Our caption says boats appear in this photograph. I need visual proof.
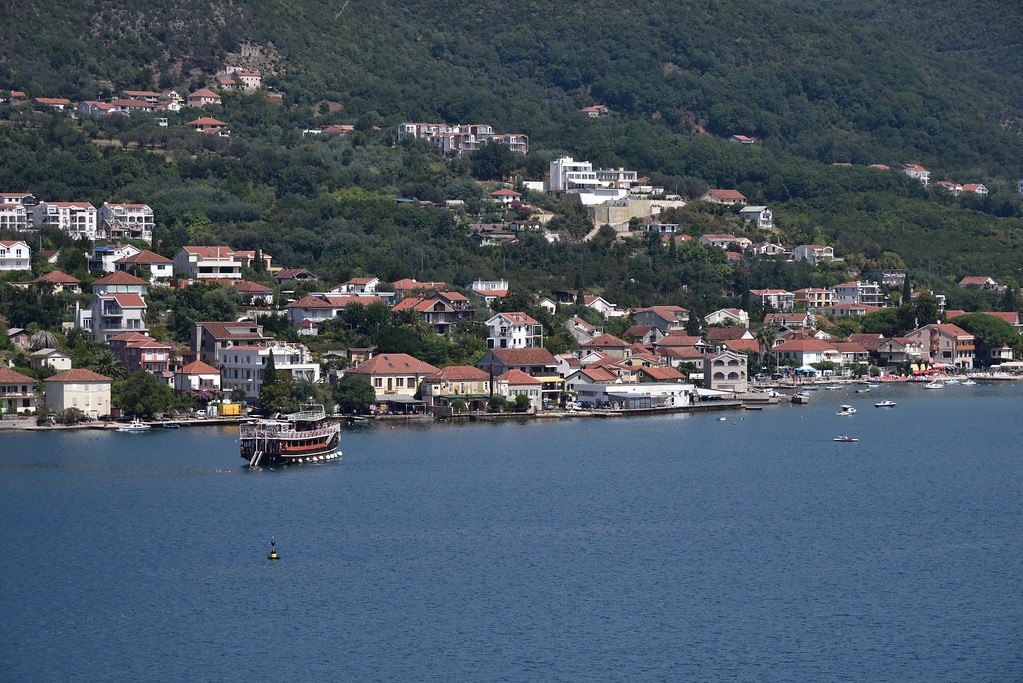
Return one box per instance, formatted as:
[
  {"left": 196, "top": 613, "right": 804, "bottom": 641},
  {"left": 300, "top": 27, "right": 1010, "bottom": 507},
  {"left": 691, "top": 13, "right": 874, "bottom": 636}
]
[
  {"left": 825, "top": 384, "right": 843, "bottom": 390},
  {"left": 834, "top": 434, "right": 859, "bottom": 442},
  {"left": 240, "top": 397, "right": 341, "bottom": 464},
  {"left": 836, "top": 404, "right": 856, "bottom": 416},
  {"left": 875, "top": 400, "right": 897, "bottom": 408},
  {"left": 923, "top": 373, "right": 976, "bottom": 389},
  {"left": 163, "top": 422, "right": 180, "bottom": 429},
  {"left": 791, "top": 380, "right": 818, "bottom": 404},
  {"left": 116, "top": 415, "right": 151, "bottom": 431},
  {"left": 868, "top": 384, "right": 880, "bottom": 388},
  {"left": 855, "top": 389, "right": 871, "bottom": 394}
]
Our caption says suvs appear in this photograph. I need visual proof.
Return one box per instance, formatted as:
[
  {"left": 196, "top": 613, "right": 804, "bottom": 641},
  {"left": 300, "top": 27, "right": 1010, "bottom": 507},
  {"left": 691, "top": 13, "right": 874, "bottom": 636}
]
[
  {"left": 47, "top": 414, "right": 57, "bottom": 425},
  {"left": 197, "top": 410, "right": 206, "bottom": 418}
]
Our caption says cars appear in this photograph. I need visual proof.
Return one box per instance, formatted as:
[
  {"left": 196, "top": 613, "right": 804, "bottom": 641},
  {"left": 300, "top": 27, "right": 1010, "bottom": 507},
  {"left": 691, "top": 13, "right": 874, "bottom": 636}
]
[
  {"left": 80, "top": 415, "right": 92, "bottom": 422},
  {"left": 755, "top": 373, "right": 784, "bottom": 381},
  {"left": 98, "top": 414, "right": 155, "bottom": 422},
  {"left": 559, "top": 400, "right": 594, "bottom": 410}
]
